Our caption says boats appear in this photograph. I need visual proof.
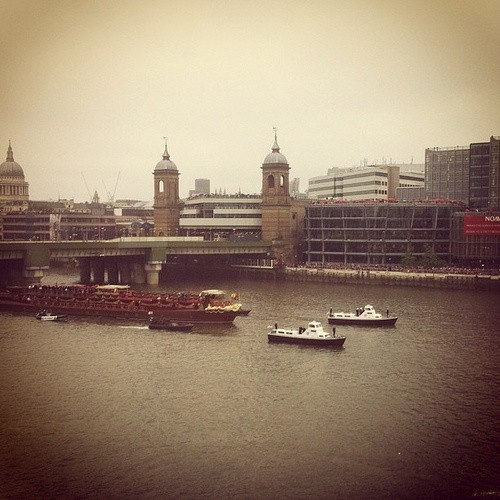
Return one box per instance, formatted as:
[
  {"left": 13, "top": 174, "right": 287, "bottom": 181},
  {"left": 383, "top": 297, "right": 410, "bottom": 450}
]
[
  {"left": 325, "top": 303, "right": 399, "bottom": 326},
  {"left": 0, "top": 282, "right": 243, "bottom": 324},
  {"left": 237, "top": 307, "right": 252, "bottom": 316},
  {"left": 33, "top": 311, "right": 69, "bottom": 321},
  {"left": 265, "top": 319, "right": 347, "bottom": 347},
  {"left": 147, "top": 311, "right": 195, "bottom": 331}
]
